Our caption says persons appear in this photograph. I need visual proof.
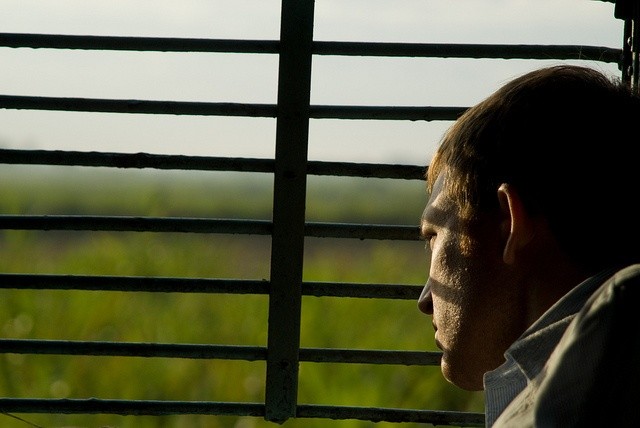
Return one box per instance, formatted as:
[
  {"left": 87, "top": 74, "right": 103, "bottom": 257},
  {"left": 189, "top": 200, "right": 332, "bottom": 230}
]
[{"left": 418, "top": 66, "right": 640, "bottom": 428}]
